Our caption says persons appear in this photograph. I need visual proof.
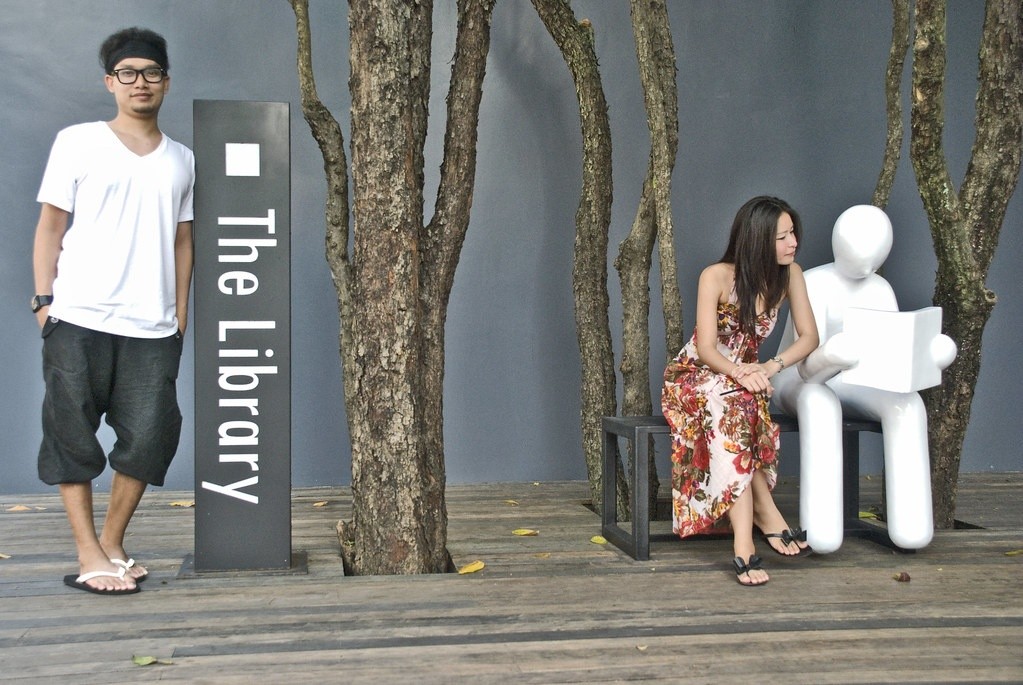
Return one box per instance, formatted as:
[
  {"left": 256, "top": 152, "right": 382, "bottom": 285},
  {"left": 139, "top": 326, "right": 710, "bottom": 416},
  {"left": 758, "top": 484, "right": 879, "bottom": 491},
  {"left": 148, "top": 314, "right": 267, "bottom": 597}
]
[
  {"left": 31, "top": 26, "right": 196, "bottom": 597},
  {"left": 770, "top": 204, "right": 957, "bottom": 555},
  {"left": 660, "top": 196, "right": 819, "bottom": 586}
]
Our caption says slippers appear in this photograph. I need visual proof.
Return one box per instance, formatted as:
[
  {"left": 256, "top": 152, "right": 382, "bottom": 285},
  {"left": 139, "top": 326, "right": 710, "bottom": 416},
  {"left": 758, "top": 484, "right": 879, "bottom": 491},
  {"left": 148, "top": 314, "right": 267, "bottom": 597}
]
[
  {"left": 64, "top": 571, "right": 140, "bottom": 594},
  {"left": 753, "top": 522, "right": 814, "bottom": 558},
  {"left": 733, "top": 556, "right": 768, "bottom": 586},
  {"left": 111, "top": 557, "right": 145, "bottom": 582}
]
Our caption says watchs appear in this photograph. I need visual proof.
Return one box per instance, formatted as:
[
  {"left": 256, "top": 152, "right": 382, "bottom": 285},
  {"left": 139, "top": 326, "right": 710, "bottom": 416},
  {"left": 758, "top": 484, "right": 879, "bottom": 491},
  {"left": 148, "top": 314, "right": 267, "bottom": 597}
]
[
  {"left": 31, "top": 295, "right": 53, "bottom": 313},
  {"left": 771, "top": 356, "right": 783, "bottom": 373}
]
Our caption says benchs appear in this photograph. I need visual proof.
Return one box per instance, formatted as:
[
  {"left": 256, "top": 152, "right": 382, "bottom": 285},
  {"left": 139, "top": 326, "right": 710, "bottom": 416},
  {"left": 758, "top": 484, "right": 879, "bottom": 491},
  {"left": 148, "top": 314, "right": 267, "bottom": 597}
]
[{"left": 601, "top": 413, "right": 915, "bottom": 563}]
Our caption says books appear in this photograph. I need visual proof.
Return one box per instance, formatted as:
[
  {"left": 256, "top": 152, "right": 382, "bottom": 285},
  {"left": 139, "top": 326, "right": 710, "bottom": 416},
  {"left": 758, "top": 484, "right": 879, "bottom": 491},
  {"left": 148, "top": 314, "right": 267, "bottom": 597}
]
[{"left": 842, "top": 305, "right": 942, "bottom": 393}]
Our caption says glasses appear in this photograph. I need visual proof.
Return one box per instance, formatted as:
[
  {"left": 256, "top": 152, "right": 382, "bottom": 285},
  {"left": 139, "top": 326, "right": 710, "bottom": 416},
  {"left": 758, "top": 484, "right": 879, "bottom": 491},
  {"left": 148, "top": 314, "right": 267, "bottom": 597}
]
[{"left": 109, "top": 66, "right": 165, "bottom": 85}]
